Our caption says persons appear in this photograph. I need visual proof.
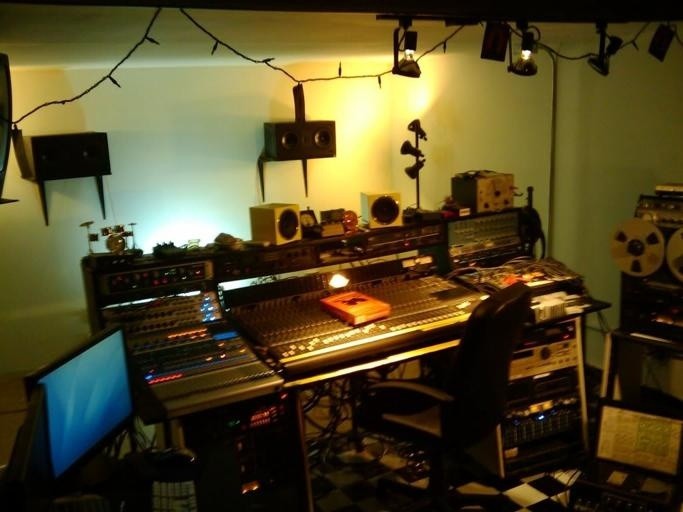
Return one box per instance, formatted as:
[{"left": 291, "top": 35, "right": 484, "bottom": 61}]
[{"left": 496, "top": 269, "right": 548, "bottom": 284}]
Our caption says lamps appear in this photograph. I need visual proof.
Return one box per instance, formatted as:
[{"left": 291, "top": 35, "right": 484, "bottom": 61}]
[{"left": 384, "top": 10, "right": 623, "bottom": 79}]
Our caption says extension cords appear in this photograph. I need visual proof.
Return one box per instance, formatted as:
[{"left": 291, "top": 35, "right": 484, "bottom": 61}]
[{"left": 337, "top": 449, "right": 375, "bottom": 463}]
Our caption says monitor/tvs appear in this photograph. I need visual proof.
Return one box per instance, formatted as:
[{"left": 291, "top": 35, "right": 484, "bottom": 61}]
[
  {"left": 593, "top": 398, "right": 683, "bottom": 478},
  {"left": 22, "top": 321, "right": 140, "bottom": 477}
]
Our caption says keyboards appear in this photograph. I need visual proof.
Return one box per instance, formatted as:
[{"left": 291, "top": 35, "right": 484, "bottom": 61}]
[{"left": 152, "top": 480, "right": 197, "bottom": 512}]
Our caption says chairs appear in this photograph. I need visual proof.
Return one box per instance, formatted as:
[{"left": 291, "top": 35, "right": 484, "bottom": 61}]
[{"left": 358, "top": 280, "right": 532, "bottom": 511}]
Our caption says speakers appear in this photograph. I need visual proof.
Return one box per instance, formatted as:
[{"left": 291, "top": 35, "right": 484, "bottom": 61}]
[
  {"left": 360, "top": 192, "right": 403, "bottom": 229},
  {"left": 248, "top": 202, "right": 302, "bottom": 246},
  {"left": 263, "top": 120, "right": 337, "bottom": 161},
  {"left": 25, "top": 129, "right": 113, "bottom": 183}
]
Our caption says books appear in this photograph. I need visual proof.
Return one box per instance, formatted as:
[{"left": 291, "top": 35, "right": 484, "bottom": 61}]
[
  {"left": 446, "top": 257, "right": 584, "bottom": 289},
  {"left": 319, "top": 290, "right": 392, "bottom": 325}
]
